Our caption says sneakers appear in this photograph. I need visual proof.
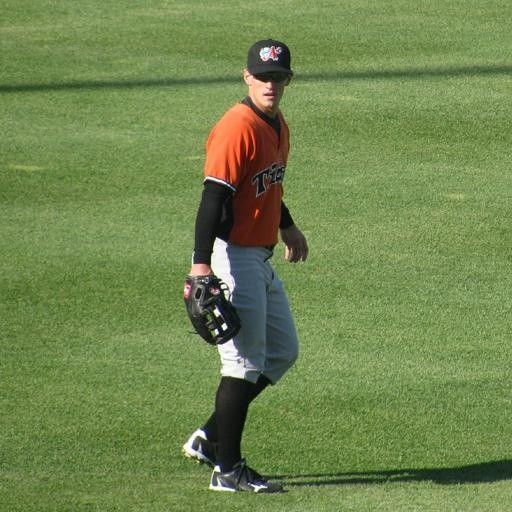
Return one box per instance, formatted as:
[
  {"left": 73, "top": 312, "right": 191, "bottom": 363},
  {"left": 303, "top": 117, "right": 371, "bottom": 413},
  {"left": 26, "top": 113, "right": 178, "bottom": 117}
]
[
  {"left": 207, "top": 457, "right": 283, "bottom": 495},
  {"left": 180, "top": 427, "right": 215, "bottom": 471}
]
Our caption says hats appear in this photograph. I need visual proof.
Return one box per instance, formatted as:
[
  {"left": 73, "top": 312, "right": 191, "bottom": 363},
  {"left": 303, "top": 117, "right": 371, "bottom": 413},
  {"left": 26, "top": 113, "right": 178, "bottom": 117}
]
[{"left": 247, "top": 39, "right": 293, "bottom": 76}]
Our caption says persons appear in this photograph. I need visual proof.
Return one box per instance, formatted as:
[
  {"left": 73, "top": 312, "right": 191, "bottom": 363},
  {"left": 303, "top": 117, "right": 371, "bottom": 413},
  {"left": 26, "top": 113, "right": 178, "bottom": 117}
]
[{"left": 181, "top": 39, "right": 309, "bottom": 493}]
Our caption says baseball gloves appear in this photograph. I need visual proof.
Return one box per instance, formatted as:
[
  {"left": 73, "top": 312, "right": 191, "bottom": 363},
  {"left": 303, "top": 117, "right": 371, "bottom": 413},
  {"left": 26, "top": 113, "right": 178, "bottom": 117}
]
[{"left": 183, "top": 276, "right": 241, "bottom": 344}]
